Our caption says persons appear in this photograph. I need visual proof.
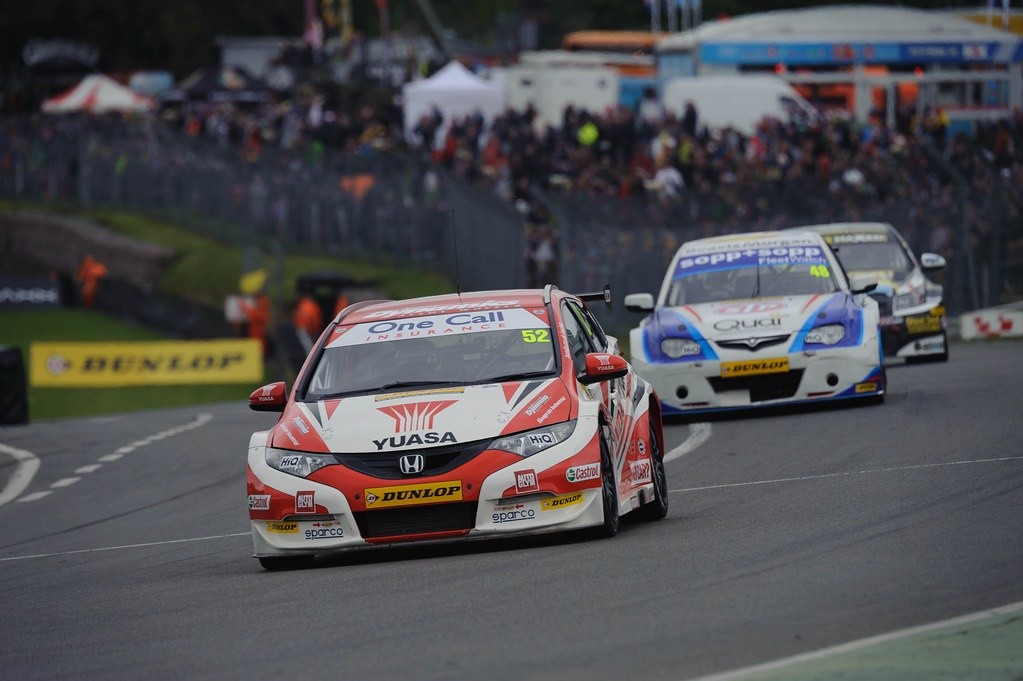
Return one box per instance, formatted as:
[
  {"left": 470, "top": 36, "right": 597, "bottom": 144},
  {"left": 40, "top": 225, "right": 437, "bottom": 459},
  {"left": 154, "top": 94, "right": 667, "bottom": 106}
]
[{"left": 0, "top": 40, "right": 1023, "bottom": 358}]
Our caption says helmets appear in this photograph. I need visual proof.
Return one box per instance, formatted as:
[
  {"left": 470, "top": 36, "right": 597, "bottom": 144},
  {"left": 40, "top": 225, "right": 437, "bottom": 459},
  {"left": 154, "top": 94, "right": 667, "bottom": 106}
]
[
  {"left": 395, "top": 339, "right": 442, "bottom": 380},
  {"left": 703, "top": 269, "right": 737, "bottom": 300}
]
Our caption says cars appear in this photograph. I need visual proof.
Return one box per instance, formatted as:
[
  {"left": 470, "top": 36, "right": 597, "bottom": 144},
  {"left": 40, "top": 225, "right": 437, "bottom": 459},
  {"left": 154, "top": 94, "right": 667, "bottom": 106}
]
[
  {"left": 246, "top": 284, "right": 668, "bottom": 572},
  {"left": 624, "top": 229, "right": 889, "bottom": 417},
  {"left": 788, "top": 221, "right": 952, "bottom": 365}
]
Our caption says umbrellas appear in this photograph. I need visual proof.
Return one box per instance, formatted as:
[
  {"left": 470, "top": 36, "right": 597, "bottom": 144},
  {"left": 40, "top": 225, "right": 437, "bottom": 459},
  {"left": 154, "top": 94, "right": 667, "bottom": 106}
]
[{"left": 40, "top": 74, "right": 162, "bottom": 114}]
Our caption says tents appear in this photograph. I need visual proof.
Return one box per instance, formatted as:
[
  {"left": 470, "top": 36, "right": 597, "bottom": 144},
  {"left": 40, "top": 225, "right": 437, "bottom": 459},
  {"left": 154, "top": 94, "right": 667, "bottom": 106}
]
[{"left": 401, "top": 59, "right": 506, "bottom": 148}]
